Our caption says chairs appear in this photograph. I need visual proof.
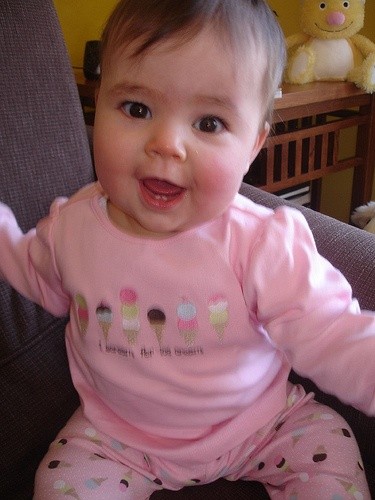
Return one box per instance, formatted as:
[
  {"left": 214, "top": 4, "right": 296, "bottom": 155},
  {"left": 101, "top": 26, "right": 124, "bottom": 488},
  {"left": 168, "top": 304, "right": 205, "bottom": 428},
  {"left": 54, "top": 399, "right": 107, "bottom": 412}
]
[{"left": 0, "top": 0, "right": 375, "bottom": 500}]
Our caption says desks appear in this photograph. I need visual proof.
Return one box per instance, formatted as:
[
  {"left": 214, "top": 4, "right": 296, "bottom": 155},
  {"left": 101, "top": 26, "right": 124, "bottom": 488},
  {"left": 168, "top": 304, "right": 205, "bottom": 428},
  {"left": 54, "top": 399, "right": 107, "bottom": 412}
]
[{"left": 73, "top": 68, "right": 375, "bottom": 230}]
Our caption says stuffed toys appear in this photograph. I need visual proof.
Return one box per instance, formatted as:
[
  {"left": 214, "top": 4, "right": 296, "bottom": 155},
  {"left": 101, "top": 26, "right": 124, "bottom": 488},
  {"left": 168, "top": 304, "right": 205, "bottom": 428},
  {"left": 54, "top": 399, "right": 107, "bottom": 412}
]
[
  {"left": 351, "top": 201, "right": 375, "bottom": 234},
  {"left": 284, "top": 0, "right": 375, "bottom": 94}
]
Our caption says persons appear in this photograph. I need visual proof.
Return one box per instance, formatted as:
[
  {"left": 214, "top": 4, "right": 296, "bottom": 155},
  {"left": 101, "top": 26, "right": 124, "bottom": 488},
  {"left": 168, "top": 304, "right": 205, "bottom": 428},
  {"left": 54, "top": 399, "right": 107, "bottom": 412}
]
[{"left": 0, "top": 0, "right": 375, "bottom": 500}]
